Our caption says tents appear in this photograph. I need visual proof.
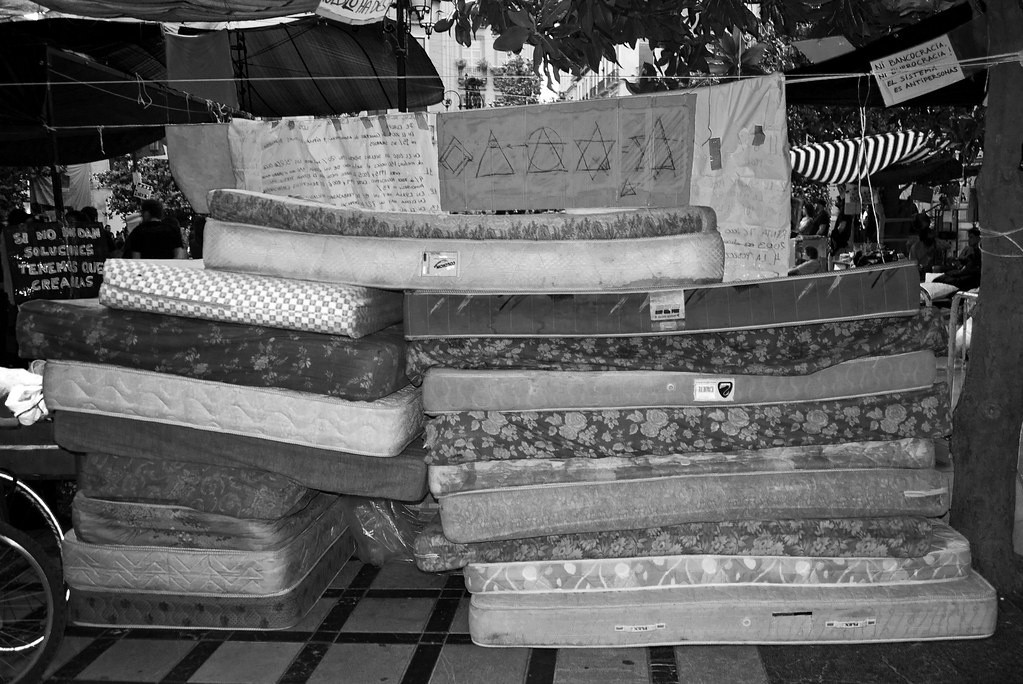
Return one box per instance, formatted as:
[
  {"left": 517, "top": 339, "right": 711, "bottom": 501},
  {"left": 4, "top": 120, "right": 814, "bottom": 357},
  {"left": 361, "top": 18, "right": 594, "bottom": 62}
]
[
  {"left": 0, "top": 0, "right": 445, "bottom": 220},
  {"left": 789, "top": 131, "right": 965, "bottom": 226}
]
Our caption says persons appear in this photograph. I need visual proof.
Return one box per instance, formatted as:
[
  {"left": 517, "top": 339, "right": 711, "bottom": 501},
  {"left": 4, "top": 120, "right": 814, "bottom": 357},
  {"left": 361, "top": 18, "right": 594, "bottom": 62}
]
[
  {"left": 790, "top": 195, "right": 982, "bottom": 307},
  {"left": 2, "top": 201, "right": 206, "bottom": 260}
]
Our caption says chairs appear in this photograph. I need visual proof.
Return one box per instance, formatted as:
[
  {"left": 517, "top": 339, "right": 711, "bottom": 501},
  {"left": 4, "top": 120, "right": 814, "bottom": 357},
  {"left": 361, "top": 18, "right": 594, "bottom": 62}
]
[{"left": 934, "top": 208, "right": 958, "bottom": 261}]
